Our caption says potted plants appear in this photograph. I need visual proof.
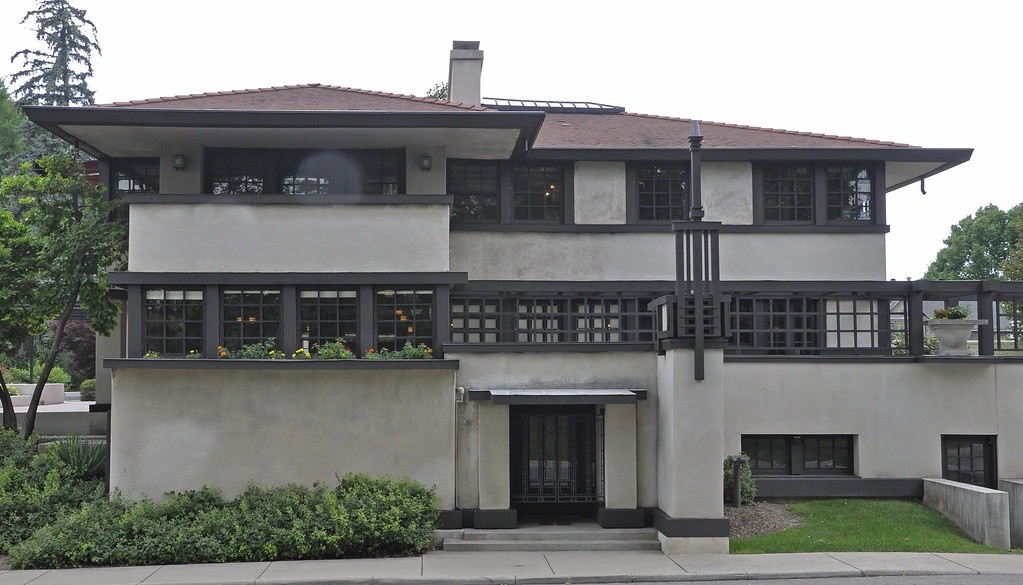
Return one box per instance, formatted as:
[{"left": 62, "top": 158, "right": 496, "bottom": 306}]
[{"left": 928, "top": 304, "right": 988, "bottom": 355}]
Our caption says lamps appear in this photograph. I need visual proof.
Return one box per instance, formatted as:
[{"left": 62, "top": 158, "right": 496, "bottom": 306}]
[
  {"left": 420, "top": 154, "right": 432, "bottom": 171},
  {"left": 172, "top": 153, "right": 185, "bottom": 170}
]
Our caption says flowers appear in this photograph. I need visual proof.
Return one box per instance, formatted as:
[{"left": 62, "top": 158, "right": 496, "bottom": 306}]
[{"left": 145, "top": 336, "right": 433, "bottom": 360}]
